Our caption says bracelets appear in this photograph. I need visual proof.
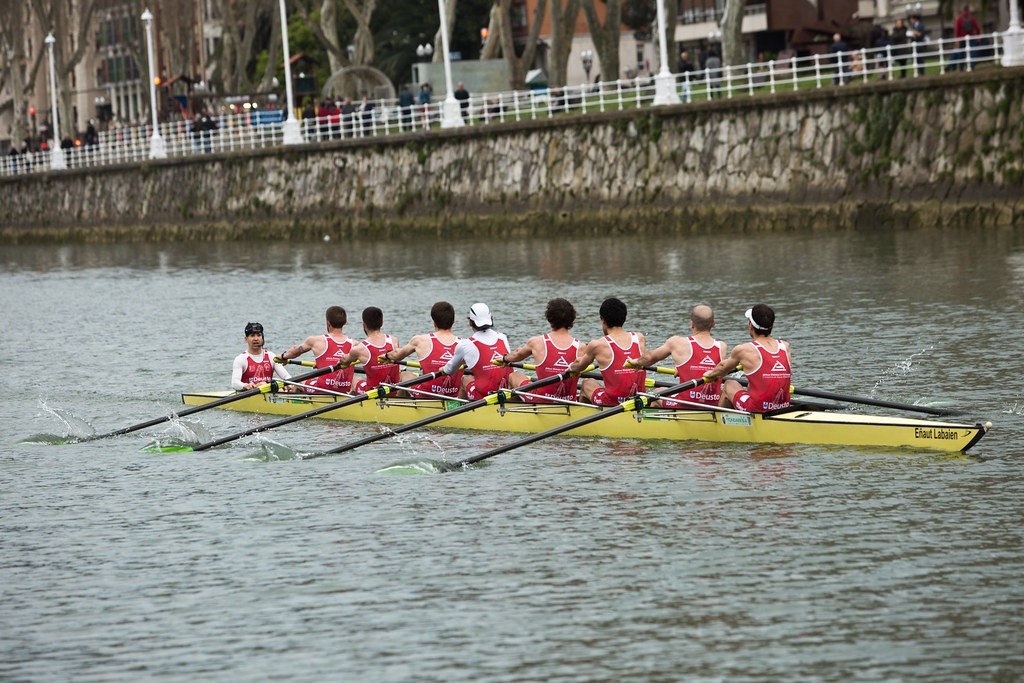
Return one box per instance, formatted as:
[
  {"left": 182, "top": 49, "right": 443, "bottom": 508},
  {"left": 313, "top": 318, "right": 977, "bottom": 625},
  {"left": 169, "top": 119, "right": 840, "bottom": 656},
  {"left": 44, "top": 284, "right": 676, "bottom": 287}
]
[
  {"left": 386, "top": 350, "right": 392, "bottom": 359},
  {"left": 503, "top": 353, "right": 508, "bottom": 363},
  {"left": 568, "top": 362, "right": 573, "bottom": 371},
  {"left": 282, "top": 351, "right": 287, "bottom": 360}
]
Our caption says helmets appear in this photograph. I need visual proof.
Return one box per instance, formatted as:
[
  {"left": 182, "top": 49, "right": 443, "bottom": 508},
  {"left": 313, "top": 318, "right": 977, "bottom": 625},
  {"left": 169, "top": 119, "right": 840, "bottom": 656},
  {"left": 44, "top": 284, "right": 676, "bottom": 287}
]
[{"left": 245, "top": 322, "right": 263, "bottom": 337}]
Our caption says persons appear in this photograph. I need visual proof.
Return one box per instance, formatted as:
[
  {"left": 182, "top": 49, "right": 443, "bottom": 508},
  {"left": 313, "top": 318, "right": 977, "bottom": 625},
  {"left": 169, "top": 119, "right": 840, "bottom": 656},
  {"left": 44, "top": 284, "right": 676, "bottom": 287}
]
[
  {"left": 547, "top": 85, "right": 564, "bottom": 113},
  {"left": 193, "top": 112, "right": 216, "bottom": 152},
  {"left": 401, "top": 82, "right": 432, "bottom": 130},
  {"left": 455, "top": 81, "right": 469, "bottom": 122},
  {"left": 302, "top": 96, "right": 372, "bottom": 140},
  {"left": 627, "top": 305, "right": 727, "bottom": 410},
  {"left": 336, "top": 307, "right": 400, "bottom": 397},
  {"left": 496, "top": 298, "right": 586, "bottom": 404},
  {"left": 377, "top": 301, "right": 466, "bottom": 398},
  {"left": 9, "top": 141, "right": 28, "bottom": 175},
  {"left": 439, "top": 303, "right": 514, "bottom": 401},
  {"left": 565, "top": 298, "right": 646, "bottom": 405},
  {"left": 830, "top": 34, "right": 863, "bottom": 84},
  {"left": 678, "top": 49, "right": 721, "bottom": 101},
  {"left": 478, "top": 98, "right": 509, "bottom": 120},
  {"left": 232, "top": 322, "right": 292, "bottom": 393},
  {"left": 85, "top": 120, "right": 95, "bottom": 150},
  {"left": 955, "top": 5, "right": 981, "bottom": 70},
  {"left": 869, "top": 16, "right": 926, "bottom": 80},
  {"left": 61, "top": 133, "right": 75, "bottom": 165},
  {"left": 276, "top": 306, "right": 359, "bottom": 393},
  {"left": 704, "top": 303, "right": 793, "bottom": 414}
]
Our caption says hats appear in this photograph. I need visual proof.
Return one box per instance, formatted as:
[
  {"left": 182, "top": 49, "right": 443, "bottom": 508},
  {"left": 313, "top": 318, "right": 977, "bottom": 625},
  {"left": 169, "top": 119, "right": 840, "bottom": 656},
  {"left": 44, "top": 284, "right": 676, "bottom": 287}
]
[
  {"left": 745, "top": 309, "right": 771, "bottom": 329},
  {"left": 470, "top": 302, "right": 493, "bottom": 326}
]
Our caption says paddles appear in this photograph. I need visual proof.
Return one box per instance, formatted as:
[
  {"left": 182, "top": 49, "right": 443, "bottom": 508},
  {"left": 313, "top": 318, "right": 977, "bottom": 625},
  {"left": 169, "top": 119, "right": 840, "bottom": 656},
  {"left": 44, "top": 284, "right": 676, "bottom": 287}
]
[
  {"left": 271, "top": 357, "right": 315, "bottom": 367},
  {"left": 373, "top": 363, "right": 743, "bottom": 475},
  {"left": 491, "top": 358, "right": 678, "bottom": 388},
  {"left": 623, "top": 360, "right": 970, "bottom": 416},
  {"left": 297, "top": 361, "right": 601, "bottom": 461},
  {"left": 55, "top": 359, "right": 359, "bottom": 445},
  {"left": 375, "top": 357, "right": 422, "bottom": 368},
  {"left": 148, "top": 362, "right": 466, "bottom": 454}
]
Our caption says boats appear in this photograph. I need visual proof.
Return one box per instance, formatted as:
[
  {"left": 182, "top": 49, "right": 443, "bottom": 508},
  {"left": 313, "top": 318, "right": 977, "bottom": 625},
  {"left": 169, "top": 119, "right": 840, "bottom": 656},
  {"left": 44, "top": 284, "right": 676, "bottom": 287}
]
[{"left": 182, "top": 391, "right": 993, "bottom": 452}]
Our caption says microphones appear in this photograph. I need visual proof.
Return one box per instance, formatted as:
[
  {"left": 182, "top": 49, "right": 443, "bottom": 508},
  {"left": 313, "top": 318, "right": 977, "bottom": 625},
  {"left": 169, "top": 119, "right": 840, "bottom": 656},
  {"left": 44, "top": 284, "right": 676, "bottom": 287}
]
[{"left": 258, "top": 334, "right": 265, "bottom": 348}]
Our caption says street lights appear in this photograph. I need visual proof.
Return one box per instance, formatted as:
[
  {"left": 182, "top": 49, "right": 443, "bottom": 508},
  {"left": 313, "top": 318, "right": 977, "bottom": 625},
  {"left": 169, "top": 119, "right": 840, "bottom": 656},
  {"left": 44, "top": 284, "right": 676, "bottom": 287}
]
[
  {"left": 43, "top": 32, "right": 68, "bottom": 170},
  {"left": 140, "top": 7, "right": 167, "bottom": 160},
  {"left": 580, "top": 50, "right": 593, "bottom": 82}
]
